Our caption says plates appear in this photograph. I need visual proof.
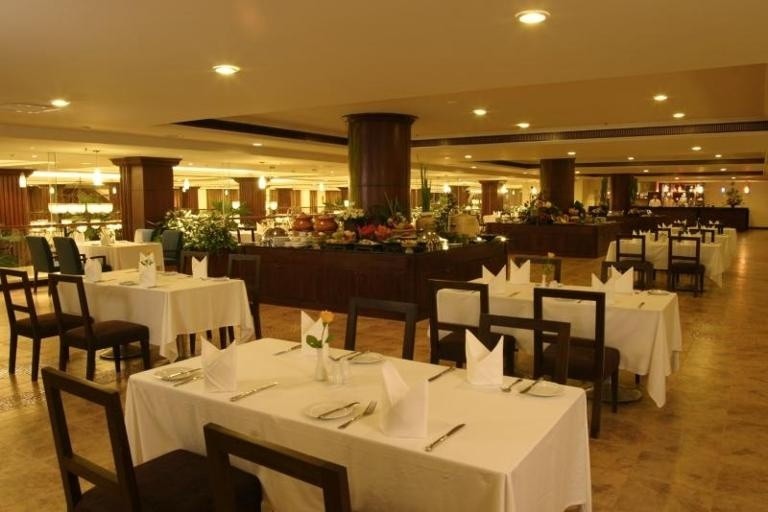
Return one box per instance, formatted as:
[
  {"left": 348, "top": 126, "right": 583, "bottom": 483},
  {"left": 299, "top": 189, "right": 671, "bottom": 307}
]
[
  {"left": 344, "top": 352, "right": 384, "bottom": 365},
  {"left": 472, "top": 239, "right": 487, "bottom": 244},
  {"left": 153, "top": 366, "right": 197, "bottom": 380},
  {"left": 520, "top": 380, "right": 563, "bottom": 398},
  {"left": 303, "top": 401, "right": 352, "bottom": 420}
]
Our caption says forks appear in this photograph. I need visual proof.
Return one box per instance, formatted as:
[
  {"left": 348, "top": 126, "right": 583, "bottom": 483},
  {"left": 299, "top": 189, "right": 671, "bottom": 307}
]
[
  {"left": 500, "top": 378, "right": 524, "bottom": 393},
  {"left": 326, "top": 351, "right": 356, "bottom": 362},
  {"left": 337, "top": 402, "right": 378, "bottom": 430}
]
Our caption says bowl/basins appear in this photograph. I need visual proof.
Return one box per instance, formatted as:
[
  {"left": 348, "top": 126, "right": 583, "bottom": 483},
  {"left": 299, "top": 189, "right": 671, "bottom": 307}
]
[{"left": 479, "top": 234, "right": 497, "bottom": 242}]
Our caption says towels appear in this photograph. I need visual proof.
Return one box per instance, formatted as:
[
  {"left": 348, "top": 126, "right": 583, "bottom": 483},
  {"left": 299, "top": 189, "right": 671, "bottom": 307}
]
[
  {"left": 197, "top": 336, "right": 238, "bottom": 394},
  {"left": 299, "top": 311, "right": 328, "bottom": 353},
  {"left": 372, "top": 355, "right": 429, "bottom": 442}
]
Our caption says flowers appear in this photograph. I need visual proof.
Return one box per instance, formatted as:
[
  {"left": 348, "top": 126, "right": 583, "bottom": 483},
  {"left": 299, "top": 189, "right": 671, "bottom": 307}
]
[
  {"left": 335, "top": 207, "right": 367, "bottom": 223},
  {"left": 306, "top": 311, "right": 336, "bottom": 348}
]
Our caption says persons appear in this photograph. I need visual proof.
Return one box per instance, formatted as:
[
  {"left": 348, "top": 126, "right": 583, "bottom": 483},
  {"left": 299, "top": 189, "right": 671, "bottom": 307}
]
[{"left": 647, "top": 195, "right": 660, "bottom": 207}]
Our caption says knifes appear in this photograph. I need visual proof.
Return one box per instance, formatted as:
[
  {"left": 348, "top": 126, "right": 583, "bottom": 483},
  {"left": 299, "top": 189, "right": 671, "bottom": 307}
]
[
  {"left": 173, "top": 375, "right": 201, "bottom": 387},
  {"left": 508, "top": 291, "right": 518, "bottom": 298},
  {"left": 428, "top": 365, "right": 455, "bottom": 384},
  {"left": 316, "top": 400, "right": 359, "bottom": 420},
  {"left": 636, "top": 301, "right": 645, "bottom": 309},
  {"left": 423, "top": 424, "right": 464, "bottom": 455},
  {"left": 228, "top": 381, "right": 278, "bottom": 403},
  {"left": 518, "top": 376, "right": 543, "bottom": 395},
  {"left": 273, "top": 342, "right": 303, "bottom": 356},
  {"left": 94, "top": 270, "right": 228, "bottom": 290},
  {"left": 168, "top": 365, "right": 201, "bottom": 379}
]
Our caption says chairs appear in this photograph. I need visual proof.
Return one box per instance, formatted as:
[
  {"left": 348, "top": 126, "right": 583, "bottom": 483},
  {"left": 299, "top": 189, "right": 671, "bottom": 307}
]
[
  {"left": 37, "top": 366, "right": 263, "bottom": 512},
  {"left": 203, "top": 422, "right": 351, "bottom": 512},
  {"left": 343, "top": 297, "right": 419, "bottom": 359}
]
